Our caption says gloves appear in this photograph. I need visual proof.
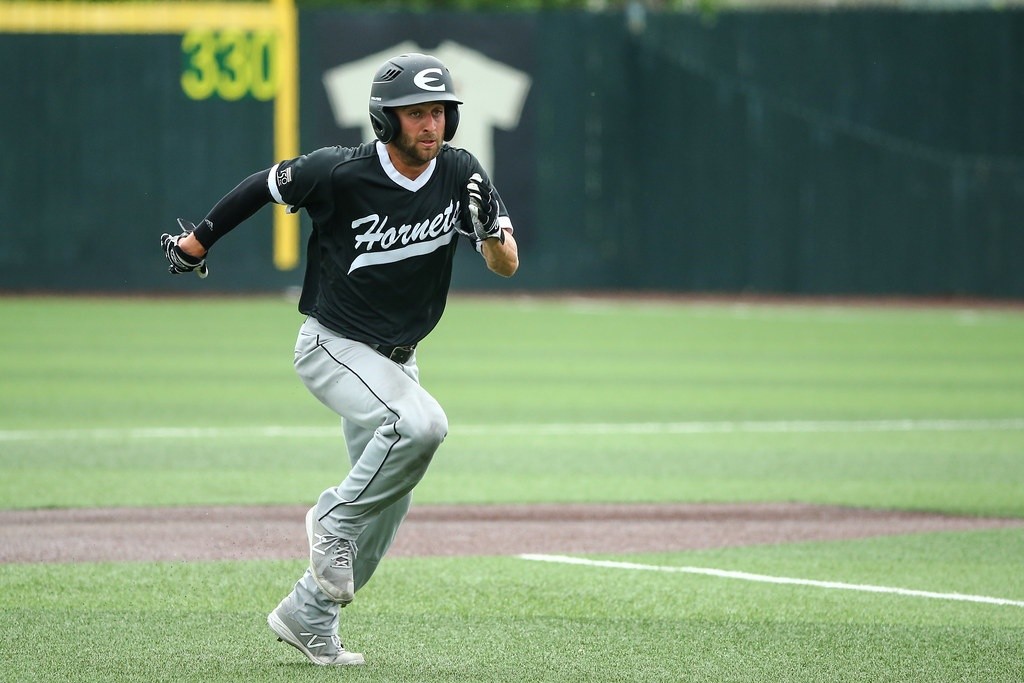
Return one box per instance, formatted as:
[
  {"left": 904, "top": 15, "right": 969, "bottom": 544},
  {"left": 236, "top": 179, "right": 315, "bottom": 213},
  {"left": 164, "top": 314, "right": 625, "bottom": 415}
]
[
  {"left": 161, "top": 218, "right": 209, "bottom": 279},
  {"left": 467, "top": 173, "right": 505, "bottom": 244}
]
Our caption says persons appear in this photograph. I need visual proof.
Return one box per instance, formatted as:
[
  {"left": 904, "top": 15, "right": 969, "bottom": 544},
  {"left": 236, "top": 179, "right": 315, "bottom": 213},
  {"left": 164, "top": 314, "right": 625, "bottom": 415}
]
[{"left": 159, "top": 53, "right": 519, "bottom": 667}]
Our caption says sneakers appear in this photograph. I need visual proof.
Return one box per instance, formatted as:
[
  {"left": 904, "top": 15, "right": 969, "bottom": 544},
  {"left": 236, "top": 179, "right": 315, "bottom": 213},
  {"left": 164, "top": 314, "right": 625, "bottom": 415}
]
[
  {"left": 306, "top": 505, "right": 354, "bottom": 601},
  {"left": 267, "top": 597, "right": 364, "bottom": 665}
]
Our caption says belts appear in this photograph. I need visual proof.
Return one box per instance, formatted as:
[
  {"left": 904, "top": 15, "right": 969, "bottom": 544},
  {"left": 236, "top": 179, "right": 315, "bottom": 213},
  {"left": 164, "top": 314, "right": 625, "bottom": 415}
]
[{"left": 370, "top": 342, "right": 417, "bottom": 364}]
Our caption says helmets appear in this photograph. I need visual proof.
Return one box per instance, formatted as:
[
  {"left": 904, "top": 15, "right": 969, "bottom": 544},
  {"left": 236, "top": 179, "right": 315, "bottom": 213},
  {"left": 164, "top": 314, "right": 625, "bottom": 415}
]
[{"left": 369, "top": 53, "right": 463, "bottom": 144}]
